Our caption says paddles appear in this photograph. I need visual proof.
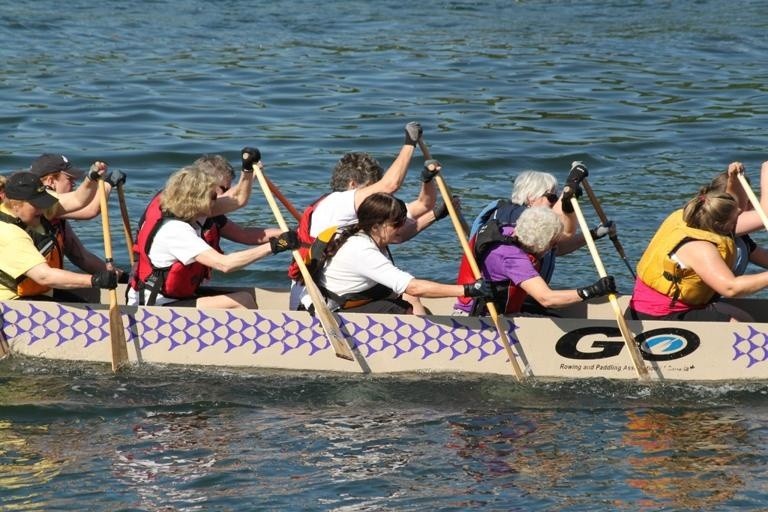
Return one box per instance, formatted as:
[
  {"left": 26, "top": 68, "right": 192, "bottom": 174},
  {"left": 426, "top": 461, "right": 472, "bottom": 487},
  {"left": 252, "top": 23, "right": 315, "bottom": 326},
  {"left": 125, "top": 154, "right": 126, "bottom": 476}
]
[
  {"left": 427, "top": 159, "right": 525, "bottom": 382},
  {"left": 92, "top": 158, "right": 130, "bottom": 373},
  {"left": 559, "top": 185, "right": 650, "bottom": 380},
  {"left": 240, "top": 153, "right": 356, "bottom": 363}
]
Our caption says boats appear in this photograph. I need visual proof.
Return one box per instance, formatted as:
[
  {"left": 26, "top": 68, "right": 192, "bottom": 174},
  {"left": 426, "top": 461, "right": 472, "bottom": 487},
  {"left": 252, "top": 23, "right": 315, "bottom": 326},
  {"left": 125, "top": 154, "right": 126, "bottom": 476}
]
[{"left": 1, "top": 276, "right": 768, "bottom": 385}]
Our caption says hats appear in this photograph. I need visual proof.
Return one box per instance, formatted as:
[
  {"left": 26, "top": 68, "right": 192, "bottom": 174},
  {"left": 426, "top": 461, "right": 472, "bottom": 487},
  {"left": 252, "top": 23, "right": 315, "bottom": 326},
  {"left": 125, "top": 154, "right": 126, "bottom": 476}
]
[
  {"left": 30, "top": 155, "right": 85, "bottom": 179},
  {"left": 4, "top": 173, "right": 58, "bottom": 209}
]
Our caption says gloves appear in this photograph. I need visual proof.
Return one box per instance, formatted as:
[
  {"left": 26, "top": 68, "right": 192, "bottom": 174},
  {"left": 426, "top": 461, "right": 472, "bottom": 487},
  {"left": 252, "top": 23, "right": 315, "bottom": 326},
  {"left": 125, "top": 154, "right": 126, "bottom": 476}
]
[
  {"left": 561, "top": 183, "right": 583, "bottom": 213},
  {"left": 464, "top": 278, "right": 494, "bottom": 297},
  {"left": 241, "top": 148, "right": 260, "bottom": 173},
  {"left": 269, "top": 231, "right": 300, "bottom": 255},
  {"left": 577, "top": 276, "right": 616, "bottom": 302},
  {"left": 433, "top": 198, "right": 460, "bottom": 221}
]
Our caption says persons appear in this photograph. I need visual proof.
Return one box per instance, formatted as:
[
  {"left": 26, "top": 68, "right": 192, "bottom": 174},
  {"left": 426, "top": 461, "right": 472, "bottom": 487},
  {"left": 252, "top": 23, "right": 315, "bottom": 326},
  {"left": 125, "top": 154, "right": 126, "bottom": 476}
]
[
  {"left": 696, "top": 162, "right": 768, "bottom": 302},
  {"left": 296, "top": 192, "right": 496, "bottom": 314},
  {"left": 125, "top": 147, "right": 301, "bottom": 309},
  {"left": 133, "top": 154, "right": 291, "bottom": 298},
  {"left": 286, "top": 121, "right": 442, "bottom": 311},
  {"left": 629, "top": 190, "right": 768, "bottom": 322},
  {"left": 468, "top": 183, "right": 616, "bottom": 317},
  {"left": 469, "top": 164, "right": 615, "bottom": 309},
  {"left": 0, "top": 160, "right": 118, "bottom": 301},
  {"left": 17, "top": 152, "right": 130, "bottom": 303}
]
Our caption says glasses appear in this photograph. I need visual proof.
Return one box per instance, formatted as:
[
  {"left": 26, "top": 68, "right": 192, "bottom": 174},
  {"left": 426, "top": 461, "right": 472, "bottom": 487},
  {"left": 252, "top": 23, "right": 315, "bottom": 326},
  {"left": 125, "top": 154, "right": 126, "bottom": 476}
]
[{"left": 543, "top": 191, "right": 558, "bottom": 203}]
